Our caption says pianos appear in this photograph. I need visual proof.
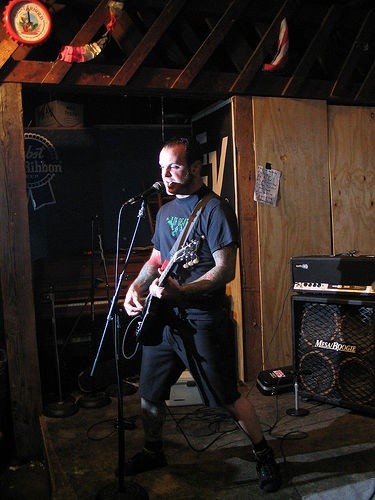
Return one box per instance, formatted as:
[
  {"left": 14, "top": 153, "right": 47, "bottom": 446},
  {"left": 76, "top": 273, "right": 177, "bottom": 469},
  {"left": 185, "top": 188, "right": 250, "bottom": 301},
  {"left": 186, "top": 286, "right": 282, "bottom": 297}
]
[{"left": 44, "top": 244, "right": 152, "bottom": 403}]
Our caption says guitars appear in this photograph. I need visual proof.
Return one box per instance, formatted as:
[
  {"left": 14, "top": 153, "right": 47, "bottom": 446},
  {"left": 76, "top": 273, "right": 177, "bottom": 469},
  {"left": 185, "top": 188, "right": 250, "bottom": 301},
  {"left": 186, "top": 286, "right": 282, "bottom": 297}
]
[{"left": 128, "top": 234, "right": 202, "bottom": 345}]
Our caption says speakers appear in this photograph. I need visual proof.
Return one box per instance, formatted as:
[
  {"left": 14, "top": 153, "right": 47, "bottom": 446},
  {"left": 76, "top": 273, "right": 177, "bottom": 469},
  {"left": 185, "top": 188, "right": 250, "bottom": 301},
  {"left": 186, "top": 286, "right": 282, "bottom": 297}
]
[{"left": 292, "top": 295, "right": 375, "bottom": 419}]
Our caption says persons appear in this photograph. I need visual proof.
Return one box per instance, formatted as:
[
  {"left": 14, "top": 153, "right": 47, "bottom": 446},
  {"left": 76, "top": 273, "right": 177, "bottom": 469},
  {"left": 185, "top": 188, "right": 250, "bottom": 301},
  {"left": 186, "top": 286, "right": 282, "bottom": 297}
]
[{"left": 114, "top": 138, "right": 278, "bottom": 490}]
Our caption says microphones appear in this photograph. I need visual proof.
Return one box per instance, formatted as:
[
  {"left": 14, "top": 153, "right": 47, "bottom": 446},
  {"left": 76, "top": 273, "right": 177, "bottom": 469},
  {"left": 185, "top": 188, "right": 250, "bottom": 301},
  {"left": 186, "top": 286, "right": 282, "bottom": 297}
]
[{"left": 128, "top": 181, "right": 164, "bottom": 205}]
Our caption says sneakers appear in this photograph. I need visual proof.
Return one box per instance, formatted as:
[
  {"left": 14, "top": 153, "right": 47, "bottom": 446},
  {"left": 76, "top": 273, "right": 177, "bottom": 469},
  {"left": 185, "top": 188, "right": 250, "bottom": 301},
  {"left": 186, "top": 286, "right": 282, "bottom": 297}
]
[
  {"left": 115, "top": 448, "right": 168, "bottom": 475},
  {"left": 252, "top": 440, "right": 280, "bottom": 492}
]
